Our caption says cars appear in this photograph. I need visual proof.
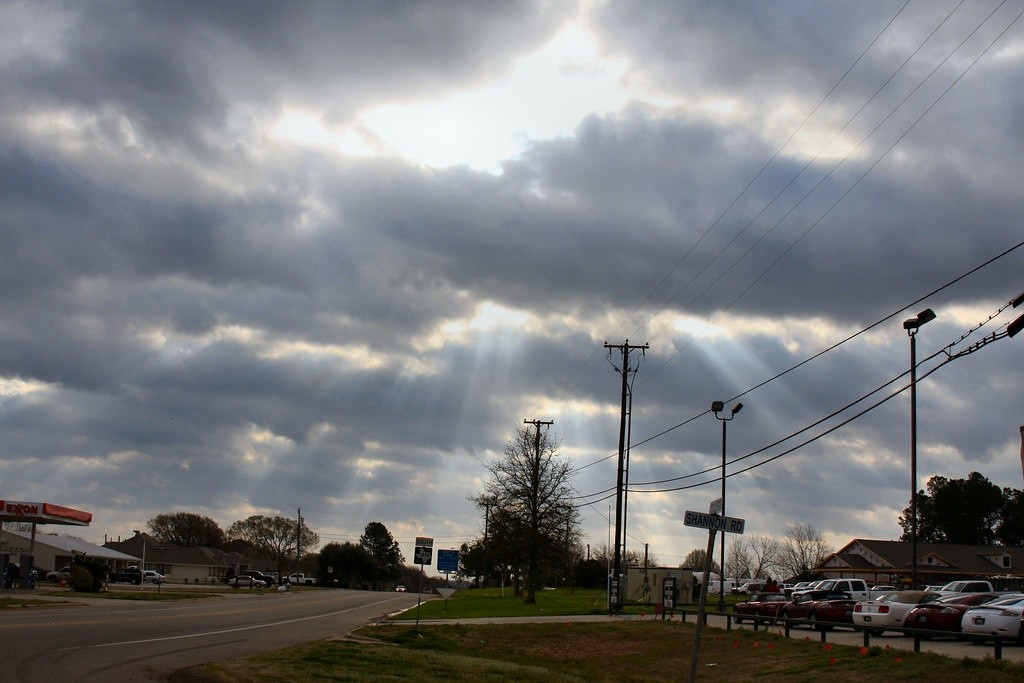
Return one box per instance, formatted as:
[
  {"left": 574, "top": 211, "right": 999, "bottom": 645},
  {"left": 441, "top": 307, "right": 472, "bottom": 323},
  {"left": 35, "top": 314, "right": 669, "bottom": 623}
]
[
  {"left": 395, "top": 586, "right": 408, "bottom": 592},
  {"left": 963, "top": 593, "right": 1024, "bottom": 646},
  {"left": 733, "top": 578, "right": 1024, "bottom": 613},
  {"left": 140, "top": 569, "right": 167, "bottom": 585},
  {"left": 732, "top": 592, "right": 793, "bottom": 626},
  {"left": 229, "top": 575, "right": 267, "bottom": 588},
  {"left": 852, "top": 589, "right": 942, "bottom": 637},
  {"left": 47, "top": 565, "right": 72, "bottom": 583},
  {"left": 904, "top": 594, "right": 1000, "bottom": 642},
  {"left": 781, "top": 589, "right": 860, "bottom": 632}
]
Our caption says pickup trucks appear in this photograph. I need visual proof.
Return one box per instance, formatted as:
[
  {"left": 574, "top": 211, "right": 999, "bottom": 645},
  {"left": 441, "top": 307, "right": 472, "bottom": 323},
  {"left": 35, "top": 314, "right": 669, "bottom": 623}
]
[{"left": 287, "top": 573, "right": 318, "bottom": 586}]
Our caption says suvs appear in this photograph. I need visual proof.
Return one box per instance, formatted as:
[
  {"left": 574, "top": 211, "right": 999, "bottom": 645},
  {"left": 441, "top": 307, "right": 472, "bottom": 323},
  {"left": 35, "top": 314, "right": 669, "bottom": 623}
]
[
  {"left": 108, "top": 567, "right": 145, "bottom": 586},
  {"left": 237, "top": 570, "right": 274, "bottom": 589}
]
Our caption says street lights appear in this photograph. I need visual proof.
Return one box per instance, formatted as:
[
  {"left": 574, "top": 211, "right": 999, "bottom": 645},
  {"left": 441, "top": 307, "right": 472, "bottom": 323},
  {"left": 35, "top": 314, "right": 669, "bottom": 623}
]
[
  {"left": 903, "top": 308, "right": 937, "bottom": 590},
  {"left": 712, "top": 400, "right": 744, "bottom": 612}
]
